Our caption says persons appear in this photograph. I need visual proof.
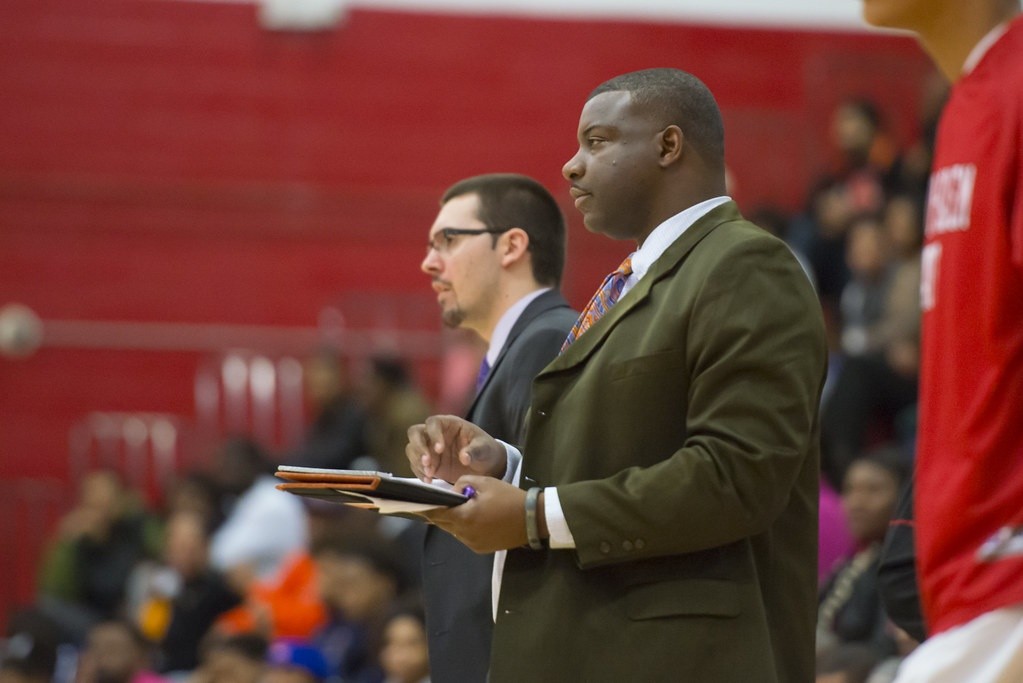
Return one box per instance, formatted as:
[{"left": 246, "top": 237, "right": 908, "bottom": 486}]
[
  {"left": 404, "top": 69, "right": 828, "bottom": 682},
  {"left": 418, "top": 174, "right": 581, "bottom": 683},
  {"left": 750, "top": 70, "right": 951, "bottom": 683},
  {"left": 0, "top": 347, "right": 432, "bottom": 683},
  {"left": 861, "top": 0, "right": 1023, "bottom": 682}
]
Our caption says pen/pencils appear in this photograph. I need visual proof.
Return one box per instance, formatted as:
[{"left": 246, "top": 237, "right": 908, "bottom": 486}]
[{"left": 463, "top": 486, "right": 475, "bottom": 498}]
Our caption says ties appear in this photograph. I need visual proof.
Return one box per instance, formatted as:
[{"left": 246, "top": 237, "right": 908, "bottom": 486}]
[{"left": 558, "top": 251, "right": 638, "bottom": 356}]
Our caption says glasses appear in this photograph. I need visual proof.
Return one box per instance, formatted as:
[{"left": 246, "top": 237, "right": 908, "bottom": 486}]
[{"left": 425, "top": 226, "right": 534, "bottom": 258}]
